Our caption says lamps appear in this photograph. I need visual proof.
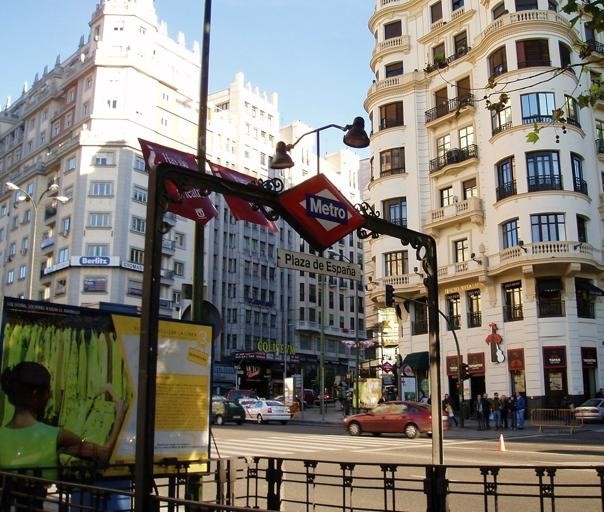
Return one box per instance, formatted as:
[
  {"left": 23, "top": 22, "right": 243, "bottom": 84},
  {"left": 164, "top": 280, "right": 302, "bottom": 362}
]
[
  {"left": 470, "top": 253, "right": 481, "bottom": 265},
  {"left": 518, "top": 240, "right": 528, "bottom": 254},
  {"left": 368, "top": 276, "right": 379, "bottom": 286},
  {"left": 413, "top": 267, "right": 423, "bottom": 278}
]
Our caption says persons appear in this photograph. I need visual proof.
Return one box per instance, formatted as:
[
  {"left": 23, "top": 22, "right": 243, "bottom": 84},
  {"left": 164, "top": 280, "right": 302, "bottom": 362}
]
[
  {"left": 416, "top": 390, "right": 428, "bottom": 403},
  {"left": 427, "top": 393, "right": 431, "bottom": 404},
  {"left": 445, "top": 403, "right": 458, "bottom": 426},
  {"left": 377, "top": 392, "right": 387, "bottom": 403},
  {"left": 475, "top": 392, "right": 525, "bottom": 431},
  {"left": 0, "top": 360, "right": 129, "bottom": 483},
  {"left": 559, "top": 394, "right": 574, "bottom": 425},
  {"left": 594, "top": 388, "right": 604, "bottom": 398},
  {"left": 441, "top": 393, "right": 451, "bottom": 410}
]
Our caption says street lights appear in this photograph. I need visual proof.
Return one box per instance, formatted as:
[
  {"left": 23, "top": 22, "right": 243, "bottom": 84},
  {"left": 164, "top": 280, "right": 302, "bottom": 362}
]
[
  {"left": 267, "top": 117, "right": 370, "bottom": 413},
  {"left": 328, "top": 249, "right": 360, "bottom": 413},
  {"left": 4, "top": 181, "right": 70, "bottom": 301}
]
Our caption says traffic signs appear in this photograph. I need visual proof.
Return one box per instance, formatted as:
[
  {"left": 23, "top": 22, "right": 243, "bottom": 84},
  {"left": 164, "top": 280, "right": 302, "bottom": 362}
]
[{"left": 278, "top": 248, "right": 362, "bottom": 282}]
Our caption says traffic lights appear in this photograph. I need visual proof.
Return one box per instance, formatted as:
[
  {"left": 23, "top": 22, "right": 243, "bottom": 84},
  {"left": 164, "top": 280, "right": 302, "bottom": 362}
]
[{"left": 386, "top": 285, "right": 395, "bottom": 307}]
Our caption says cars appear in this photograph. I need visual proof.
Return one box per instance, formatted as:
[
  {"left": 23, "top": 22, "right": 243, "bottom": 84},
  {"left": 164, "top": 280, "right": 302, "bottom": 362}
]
[
  {"left": 274, "top": 388, "right": 334, "bottom": 411},
  {"left": 211, "top": 395, "right": 245, "bottom": 425},
  {"left": 574, "top": 398, "right": 604, "bottom": 423},
  {"left": 340, "top": 400, "right": 449, "bottom": 438},
  {"left": 226, "top": 387, "right": 291, "bottom": 424}
]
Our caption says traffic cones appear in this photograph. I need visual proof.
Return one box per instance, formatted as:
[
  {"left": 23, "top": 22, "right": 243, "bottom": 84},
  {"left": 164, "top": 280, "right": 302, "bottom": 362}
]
[{"left": 497, "top": 433, "right": 506, "bottom": 451}]
